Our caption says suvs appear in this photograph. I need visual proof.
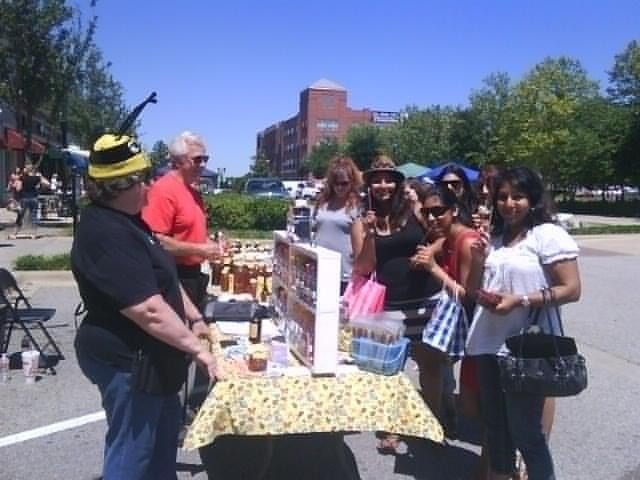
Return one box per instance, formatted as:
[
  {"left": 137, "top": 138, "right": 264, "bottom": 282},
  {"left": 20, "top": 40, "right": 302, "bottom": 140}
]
[{"left": 238, "top": 176, "right": 296, "bottom": 207}]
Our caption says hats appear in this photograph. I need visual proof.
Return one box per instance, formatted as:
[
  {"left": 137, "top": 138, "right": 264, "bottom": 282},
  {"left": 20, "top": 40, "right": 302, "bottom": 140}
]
[
  {"left": 87, "top": 134, "right": 152, "bottom": 180},
  {"left": 363, "top": 155, "right": 404, "bottom": 184},
  {"left": 421, "top": 177, "right": 435, "bottom": 185}
]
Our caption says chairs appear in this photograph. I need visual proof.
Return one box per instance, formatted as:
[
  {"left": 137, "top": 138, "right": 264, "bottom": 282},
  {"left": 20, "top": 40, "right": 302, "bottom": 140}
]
[{"left": 0, "top": 267, "right": 66, "bottom": 375}]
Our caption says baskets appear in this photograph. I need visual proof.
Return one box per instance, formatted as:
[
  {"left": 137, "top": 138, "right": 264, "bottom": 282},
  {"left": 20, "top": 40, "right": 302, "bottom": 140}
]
[{"left": 350, "top": 336, "right": 411, "bottom": 375}]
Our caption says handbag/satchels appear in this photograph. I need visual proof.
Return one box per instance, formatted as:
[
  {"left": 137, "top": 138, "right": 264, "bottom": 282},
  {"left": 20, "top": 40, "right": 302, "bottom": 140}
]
[
  {"left": 131, "top": 346, "right": 179, "bottom": 396},
  {"left": 496, "top": 327, "right": 588, "bottom": 397},
  {"left": 421, "top": 292, "right": 470, "bottom": 361},
  {"left": 341, "top": 275, "right": 388, "bottom": 322}
]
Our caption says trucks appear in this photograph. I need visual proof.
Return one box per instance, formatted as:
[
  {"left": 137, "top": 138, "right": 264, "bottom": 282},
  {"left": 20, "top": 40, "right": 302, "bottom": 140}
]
[{"left": 280, "top": 180, "right": 321, "bottom": 200}]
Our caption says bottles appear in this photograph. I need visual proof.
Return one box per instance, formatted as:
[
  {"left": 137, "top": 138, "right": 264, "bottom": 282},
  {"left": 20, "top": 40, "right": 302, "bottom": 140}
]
[
  {"left": 464, "top": 288, "right": 502, "bottom": 307},
  {"left": 268, "top": 241, "right": 318, "bottom": 362},
  {"left": 245, "top": 302, "right": 262, "bottom": 341},
  {"left": 205, "top": 231, "right": 271, "bottom": 297},
  {"left": 478, "top": 205, "right": 490, "bottom": 227},
  {"left": 367, "top": 211, "right": 377, "bottom": 235}
]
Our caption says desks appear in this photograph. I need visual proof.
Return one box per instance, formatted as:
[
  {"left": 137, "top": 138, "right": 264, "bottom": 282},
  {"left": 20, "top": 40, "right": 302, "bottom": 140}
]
[{"left": 182, "top": 238, "right": 445, "bottom": 480}]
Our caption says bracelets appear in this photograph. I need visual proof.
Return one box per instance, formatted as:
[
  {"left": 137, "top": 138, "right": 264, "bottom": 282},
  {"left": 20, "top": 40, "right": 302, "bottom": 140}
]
[{"left": 191, "top": 316, "right": 204, "bottom": 324}]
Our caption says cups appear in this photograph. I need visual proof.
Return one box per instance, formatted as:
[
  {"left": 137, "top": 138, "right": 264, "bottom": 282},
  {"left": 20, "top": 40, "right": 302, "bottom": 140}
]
[{"left": 21, "top": 350, "right": 41, "bottom": 384}]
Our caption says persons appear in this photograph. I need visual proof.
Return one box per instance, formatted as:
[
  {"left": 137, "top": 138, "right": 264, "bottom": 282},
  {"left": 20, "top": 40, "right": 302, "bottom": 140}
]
[
  {"left": 141, "top": 132, "right": 229, "bottom": 445},
  {"left": 463, "top": 167, "right": 581, "bottom": 479},
  {"left": 7, "top": 162, "right": 65, "bottom": 234},
  {"left": 313, "top": 154, "right": 556, "bottom": 480},
  {"left": 70, "top": 133, "right": 220, "bottom": 478}
]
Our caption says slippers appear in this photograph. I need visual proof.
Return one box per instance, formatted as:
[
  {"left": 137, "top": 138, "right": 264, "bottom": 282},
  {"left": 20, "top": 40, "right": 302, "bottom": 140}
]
[{"left": 382, "top": 440, "right": 400, "bottom": 454}]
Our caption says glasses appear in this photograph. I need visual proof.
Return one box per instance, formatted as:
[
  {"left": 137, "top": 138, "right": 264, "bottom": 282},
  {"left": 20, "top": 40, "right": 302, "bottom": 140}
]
[
  {"left": 187, "top": 156, "right": 209, "bottom": 164},
  {"left": 420, "top": 206, "right": 450, "bottom": 218},
  {"left": 440, "top": 180, "right": 462, "bottom": 192}
]
